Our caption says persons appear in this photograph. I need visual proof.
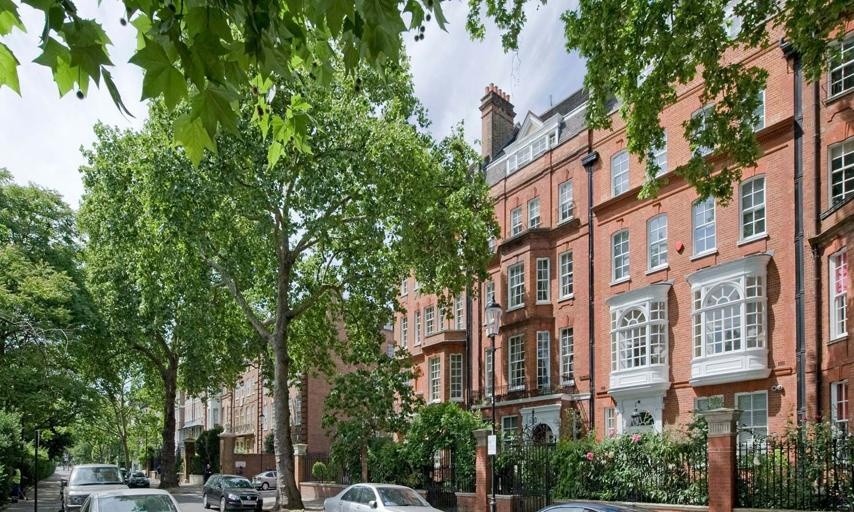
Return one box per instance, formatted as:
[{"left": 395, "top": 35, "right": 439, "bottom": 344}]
[
  {"left": 9, "top": 465, "right": 21, "bottom": 503},
  {"left": 203, "top": 463, "right": 212, "bottom": 482},
  {"left": 234, "top": 465, "right": 243, "bottom": 475}
]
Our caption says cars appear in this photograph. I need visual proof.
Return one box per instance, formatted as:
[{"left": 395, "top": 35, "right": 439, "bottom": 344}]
[
  {"left": 59, "top": 464, "right": 182, "bottom": 512},
  {"left": 323, "top": 483, "right": 445, "bottom": 512},
  {"left": 535, "top": 502, "right": 659, "bottom": 512},
  {"left": 202, "top": 471, "right": 277, "bottom": 512}
]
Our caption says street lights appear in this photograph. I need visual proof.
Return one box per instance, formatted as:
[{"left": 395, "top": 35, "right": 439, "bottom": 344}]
[
  {"left": 144, "top": 422, "right": 150, "bottom": 477},
  {"left": 484, "top": 295, "right": 503, "bottom": 512}
]
